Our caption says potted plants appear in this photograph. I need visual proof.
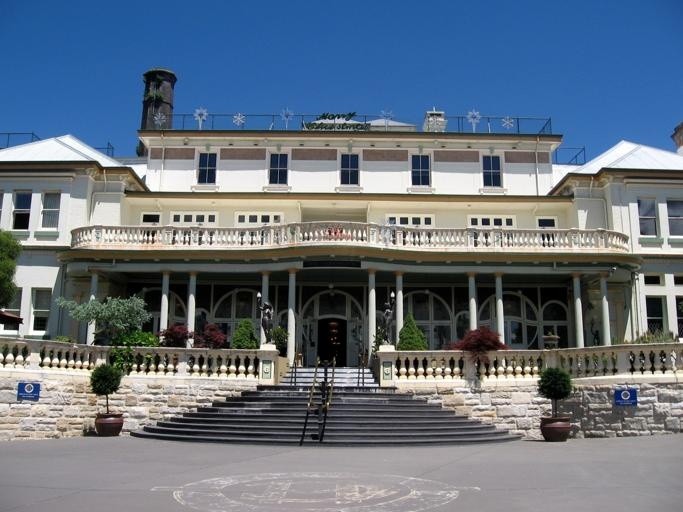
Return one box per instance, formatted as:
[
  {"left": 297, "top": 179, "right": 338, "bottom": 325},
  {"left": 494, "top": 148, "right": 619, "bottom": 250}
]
[
  {"left": 537, "top": 368, "right": 573, "bottom": 441},
  {"left": 89, "top": 362, "right": 123, "bottom": 435}
]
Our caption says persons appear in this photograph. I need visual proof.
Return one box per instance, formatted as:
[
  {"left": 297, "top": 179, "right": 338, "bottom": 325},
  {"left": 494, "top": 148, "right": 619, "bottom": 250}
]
[
  {"left": 257, "top": 299, "right": 273, "bottom": 343},
  {"left": 383, "top": 299, "right": 396, "bottom": 343}
]
